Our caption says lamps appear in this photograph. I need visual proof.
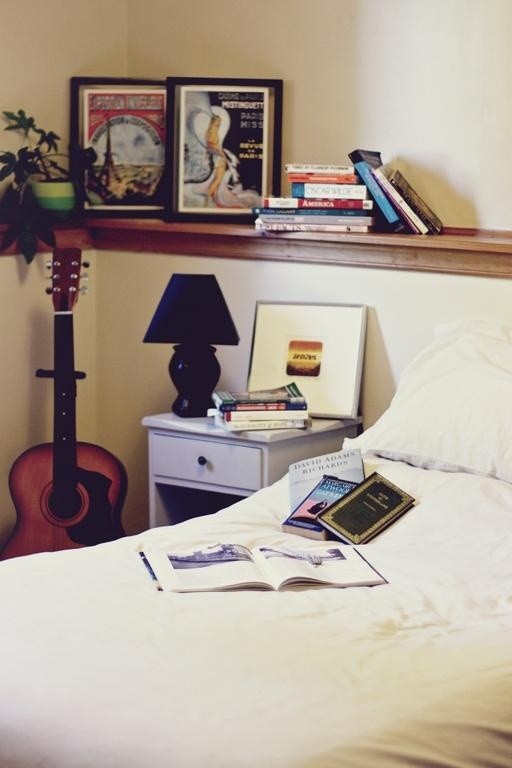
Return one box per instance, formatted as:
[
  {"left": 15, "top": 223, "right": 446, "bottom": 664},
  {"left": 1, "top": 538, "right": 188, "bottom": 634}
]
[{"left": 142, "top": 273, "right": 240, "bottom": 420}]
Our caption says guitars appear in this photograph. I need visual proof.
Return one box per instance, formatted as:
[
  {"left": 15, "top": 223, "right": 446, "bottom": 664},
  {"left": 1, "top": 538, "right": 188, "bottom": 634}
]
[{"left": 1, "top": 246, "right": 130, "bottom": 563}]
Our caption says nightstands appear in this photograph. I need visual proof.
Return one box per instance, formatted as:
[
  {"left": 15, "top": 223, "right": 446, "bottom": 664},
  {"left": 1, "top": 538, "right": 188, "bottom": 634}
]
[{"left": 138, "top": 413, "right": 365, "bottom": 530}]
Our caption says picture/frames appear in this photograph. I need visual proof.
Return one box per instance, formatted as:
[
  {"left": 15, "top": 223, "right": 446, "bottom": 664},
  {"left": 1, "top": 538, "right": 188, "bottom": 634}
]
[
  {"left": 166, "top": 77, "right": 285, "bottom": 223},
  {"left": 243, "top": 296, "right": 369, "bottom": 421},
  {"left": 68, "top": 75, "right": 169, "bottom": 219}
]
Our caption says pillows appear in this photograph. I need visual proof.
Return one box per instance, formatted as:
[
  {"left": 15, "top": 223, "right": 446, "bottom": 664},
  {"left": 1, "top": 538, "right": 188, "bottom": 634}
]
[{"left": 342, "top": 327, "right": 511, "bottom": 482}]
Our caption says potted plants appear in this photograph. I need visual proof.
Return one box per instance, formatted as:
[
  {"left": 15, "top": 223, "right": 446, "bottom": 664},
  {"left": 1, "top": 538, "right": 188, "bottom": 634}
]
[{"left": 0, "top": 106, "right": 76, "bottom": 266}]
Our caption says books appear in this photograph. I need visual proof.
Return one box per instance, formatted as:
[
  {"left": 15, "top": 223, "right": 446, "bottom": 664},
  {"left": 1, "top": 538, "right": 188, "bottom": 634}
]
[
  {"left": 374, "top": 163, "right": 429, "bottom": 235},
  {"left": 315, "top": 471, "right": 420, "bottom": 545},
  {"left": 291, "top": 184, "right": 367, "bottom": 199},
  {"left": 371, "top": 170, "right": 419, "bottom": 234},
  {"left": 348, "top": 150, "right": 405, "bottom": 233},
  {"left": 136, "top": 540, "right": 390, "bottom": 593},
  {"left": 284, "top": 164, "right": 356, "bottom": 175},
  {"left": 260, "top": 198, "right": 373, "bottom": 210},
  {"left": 281, "top": 474, "right": 360, "bottom": 541},
  {"left": 215, "top": 419, "right": 307, "bottom": 432},
  {"left": 259, "top": 216, "right": 372, "bottom": 226},
  {"left": 210, "top": 381, "right": 305, "bottom": 404},
  {"left": 288, "top": 173, "right": 360, "bottom": 184},
  {"left": 251, "top": 207, "right": 368, "bottom": 216},
  {"left": 263, "top": 224, "right": 368, "bottom": 233},
  {"left": 206, "top": 409, "right": 308, "bottom": 422},
  {"left": 388, "top": 169, "right": 442, "bottom": 235},
  {"left": 214, "top": 402, "right": 308, "bottom": 412}
]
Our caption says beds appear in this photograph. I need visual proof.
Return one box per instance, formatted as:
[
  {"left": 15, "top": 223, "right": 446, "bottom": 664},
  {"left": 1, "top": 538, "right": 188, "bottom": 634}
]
[{"left": 0, "top": 325, "right": 512, "bottom": 768}]
